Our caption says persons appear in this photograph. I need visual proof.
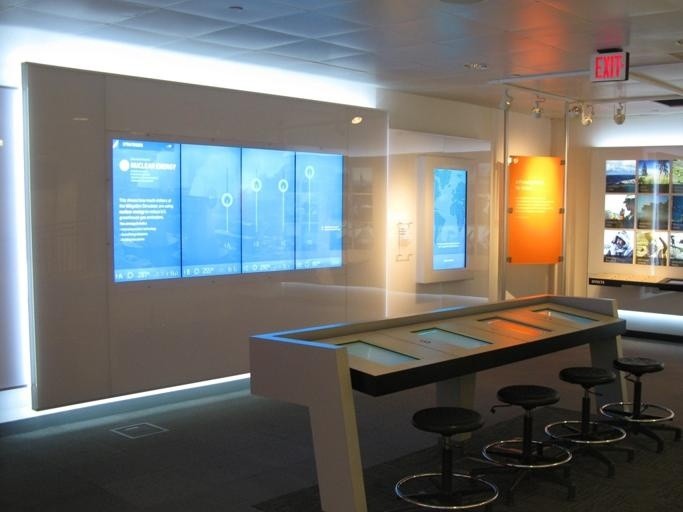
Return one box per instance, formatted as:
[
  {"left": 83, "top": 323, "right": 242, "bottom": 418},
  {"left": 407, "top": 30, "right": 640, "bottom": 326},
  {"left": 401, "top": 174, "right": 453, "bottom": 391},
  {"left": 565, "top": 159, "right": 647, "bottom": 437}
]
[
  {"left": 619, "top": 195, "right": 634, "bottom": 227},
  {"left": 605, "top": 229, "right": 633, "bottom": 262}
]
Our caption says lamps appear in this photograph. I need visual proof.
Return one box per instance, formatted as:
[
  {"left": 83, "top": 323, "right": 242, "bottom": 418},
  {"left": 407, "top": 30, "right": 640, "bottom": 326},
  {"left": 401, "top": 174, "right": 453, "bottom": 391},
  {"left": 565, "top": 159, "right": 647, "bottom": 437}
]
[
  {"left": 498, "top": 87, "right": 544, "bottom": 120},
  {"left": 569, "top": 102, "right": 627, "bottom": 127}
]
